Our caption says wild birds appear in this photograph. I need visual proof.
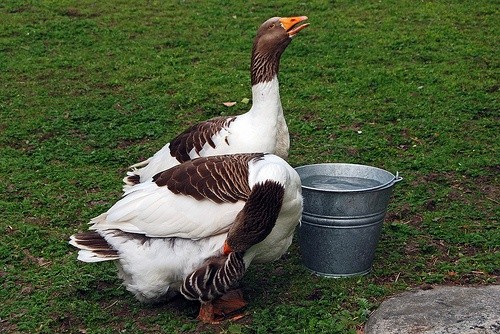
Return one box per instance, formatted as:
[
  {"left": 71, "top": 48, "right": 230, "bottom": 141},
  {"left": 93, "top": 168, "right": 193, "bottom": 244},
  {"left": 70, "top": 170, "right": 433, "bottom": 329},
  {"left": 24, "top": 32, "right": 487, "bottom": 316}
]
[
  {"left": 68, "top": 152, "right": 305, "bottom": 327},
  {"left": 121, "top": 15, "right": 311, "bottom": 205}
]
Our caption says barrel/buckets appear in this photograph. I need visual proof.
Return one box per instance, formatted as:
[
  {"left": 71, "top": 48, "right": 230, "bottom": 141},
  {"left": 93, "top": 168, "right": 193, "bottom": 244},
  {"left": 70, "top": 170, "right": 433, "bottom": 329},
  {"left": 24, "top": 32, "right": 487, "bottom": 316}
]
[{"left": 288, "top": 163, "right": 404, "bottom": 278}]
